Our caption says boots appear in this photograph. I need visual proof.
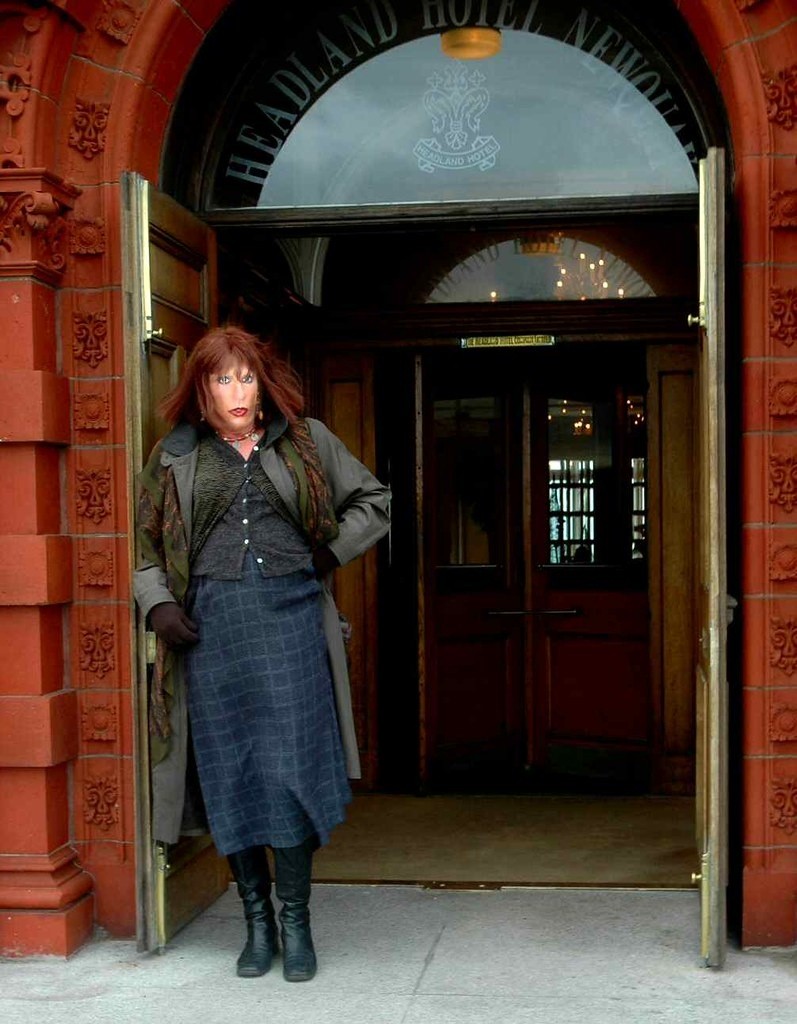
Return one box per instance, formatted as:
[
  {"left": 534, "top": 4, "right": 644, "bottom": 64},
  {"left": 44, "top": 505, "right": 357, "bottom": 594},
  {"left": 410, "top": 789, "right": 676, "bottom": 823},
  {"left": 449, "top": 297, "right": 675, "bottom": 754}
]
[
  {"left": 273, "top": 839, "right": 317, "bottom": 982},
  {"left": 227, "top": 842, "right": 279, "bottom": 977}
]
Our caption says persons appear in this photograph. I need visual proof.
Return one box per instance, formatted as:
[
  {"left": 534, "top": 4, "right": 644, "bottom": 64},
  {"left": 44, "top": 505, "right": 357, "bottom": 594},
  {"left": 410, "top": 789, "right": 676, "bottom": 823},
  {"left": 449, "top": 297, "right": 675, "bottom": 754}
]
[{"left": 131, "top": 325, "right": 390, "bottom": 982}]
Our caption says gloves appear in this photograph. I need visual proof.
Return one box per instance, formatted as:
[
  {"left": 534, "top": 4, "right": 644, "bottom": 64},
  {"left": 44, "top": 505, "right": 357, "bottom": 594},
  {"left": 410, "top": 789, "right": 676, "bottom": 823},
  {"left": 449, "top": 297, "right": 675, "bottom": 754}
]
[
  {"left": 312, "top": 543, "right": 341, "bottom": 582},
  {"left": 151, "top": 601, "right": 199, "bottom": 653}
]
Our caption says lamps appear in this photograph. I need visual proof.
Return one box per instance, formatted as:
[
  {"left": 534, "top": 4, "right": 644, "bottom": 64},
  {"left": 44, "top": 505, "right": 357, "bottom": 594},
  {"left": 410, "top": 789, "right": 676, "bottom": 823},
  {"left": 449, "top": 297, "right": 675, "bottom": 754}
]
[
  {"left": 522, "top": 232, "right": 563, "bottom": 255},
  {"left": 441, "top": 27, "right": 503, "bottom": 59}
]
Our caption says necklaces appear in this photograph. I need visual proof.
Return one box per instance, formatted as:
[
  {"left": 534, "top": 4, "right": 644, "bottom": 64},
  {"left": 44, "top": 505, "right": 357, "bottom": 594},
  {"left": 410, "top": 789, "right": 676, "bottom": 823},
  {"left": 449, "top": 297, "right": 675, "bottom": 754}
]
[{"left": 222, "top": 427, "right": 259, "bottom": 449}]
